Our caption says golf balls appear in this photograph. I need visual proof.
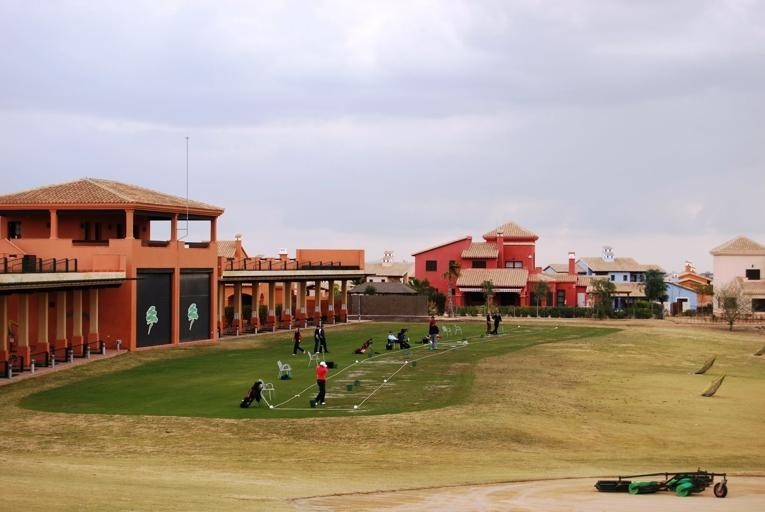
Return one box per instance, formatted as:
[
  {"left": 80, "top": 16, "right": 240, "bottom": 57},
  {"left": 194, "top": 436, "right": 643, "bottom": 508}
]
[
  {"left": 354, "top": 405, "right": 358, "bottom": 409},
  {"left": 384, "top": 380, "right": 387, "bottom": 383},
  {"left": 356, "top": 360, "right": 358, "bottom": 363}
]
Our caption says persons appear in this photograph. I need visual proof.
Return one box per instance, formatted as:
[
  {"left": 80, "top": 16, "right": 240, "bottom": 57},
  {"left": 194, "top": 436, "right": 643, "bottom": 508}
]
[
  {"left": 318, "top": 325, "right": 329, "bottom": 353},
  {"left": 490, "top": 312, "right": 502, "bottom": 334},
  {"left": 313, "top": 361, "right": 327, "bottom": 406},
  {"left": 291, "top": 327, "right": 306, "bottom": 356},
  {"left": 487, "top": 314, "right": 493, "bottom": 335},
  {"left": 313, "top": 324, "right": 321, "bottom": 354},
  {"left": 430, "top": 316, "right": 437, "bottom": 326},
  {"left": 429, "top": 322, "right": 439, "bottom": 350},
  {"left": 387, "top": 331, "right": 399, "bottom": 345}
]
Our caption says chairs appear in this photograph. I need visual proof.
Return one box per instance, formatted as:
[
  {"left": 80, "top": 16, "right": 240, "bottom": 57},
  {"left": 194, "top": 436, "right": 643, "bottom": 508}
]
[
  {"left": 308, "top": 352, "right": 320, "bottom": 367},
  {"left": 278, "top": 360, "right": 291, "bottom": 378},
  {"left": 387, "top": 338, "right": 395, "bottom": 349},
  {"left": 442, "top": 326, "right": 452, "bottom": 337},
  {"left": 455, "top": 324, "right": 462, "bottom": 335},
  {"left": 259, "top": 379, "right": 275, "bottom": 400}
]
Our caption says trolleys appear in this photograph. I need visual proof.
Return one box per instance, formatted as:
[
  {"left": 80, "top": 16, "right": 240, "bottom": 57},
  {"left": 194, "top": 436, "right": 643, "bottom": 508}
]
[
  {"left": 355, "top": 337, "right": 374, "bottom": 354},
  {"left": 240, "top": 381, "right": 263, "bottom": 408}
]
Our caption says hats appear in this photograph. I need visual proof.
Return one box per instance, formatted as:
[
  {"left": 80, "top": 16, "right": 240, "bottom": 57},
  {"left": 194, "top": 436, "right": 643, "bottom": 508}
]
[{"left": 320, "top": 361, "right": 327, "bottom": 367}]
